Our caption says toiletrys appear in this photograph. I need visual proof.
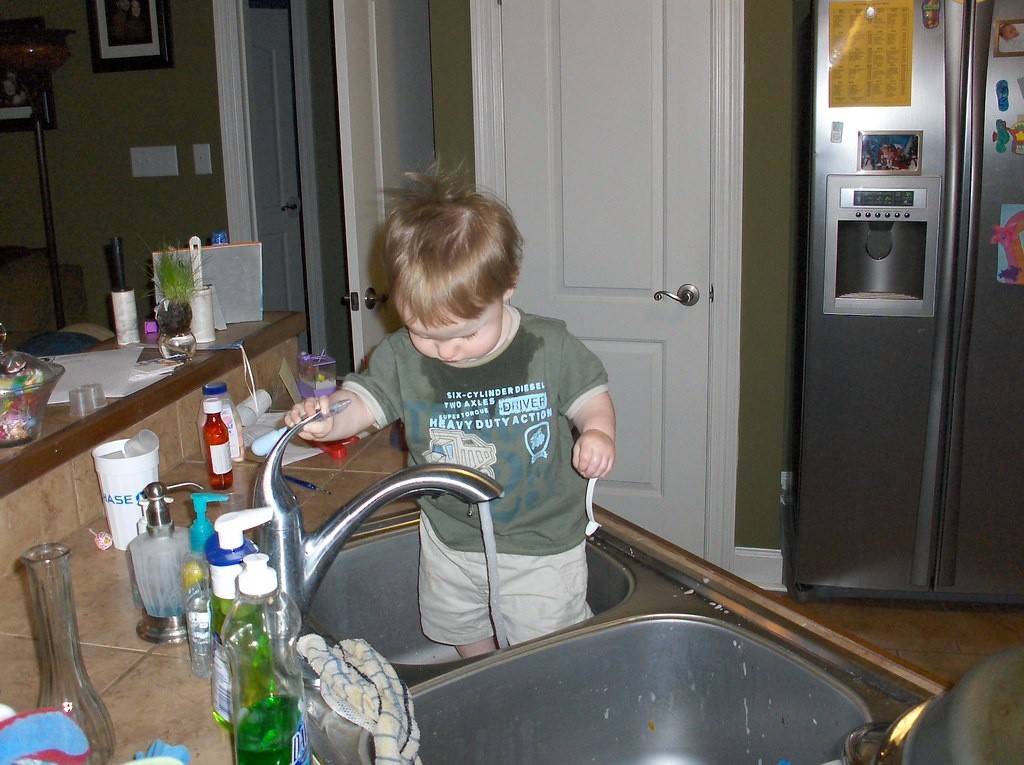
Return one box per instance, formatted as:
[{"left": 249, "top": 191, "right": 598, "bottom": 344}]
[{"left": 197, "top": 381, "right": 245, "bottom": 462}]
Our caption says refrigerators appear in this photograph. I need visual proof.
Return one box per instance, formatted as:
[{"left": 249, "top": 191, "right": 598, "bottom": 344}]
[{"left": 791, "top": 0, "right": 1024, "bottom": 617}]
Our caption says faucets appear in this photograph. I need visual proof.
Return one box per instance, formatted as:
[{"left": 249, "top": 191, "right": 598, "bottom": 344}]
[{"left": 252, "top": 394, "right": 503, "bottom": 628}]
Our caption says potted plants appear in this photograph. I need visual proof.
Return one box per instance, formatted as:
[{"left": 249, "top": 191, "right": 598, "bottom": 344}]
[{"left": 125, "top": 231, "right": 215, "bottom": 361}]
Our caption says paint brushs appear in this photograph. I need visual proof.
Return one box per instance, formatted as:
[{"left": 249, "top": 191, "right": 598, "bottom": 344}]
[{"left": 282, "top": 473, "right": 332, "bottom": 496}]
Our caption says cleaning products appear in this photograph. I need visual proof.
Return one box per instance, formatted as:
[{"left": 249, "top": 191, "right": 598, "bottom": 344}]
[{"left": 174, "top": 486, "right": 313, "bottom": 765}]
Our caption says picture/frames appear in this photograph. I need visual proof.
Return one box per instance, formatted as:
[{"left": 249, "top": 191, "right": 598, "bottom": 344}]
[
  {"left": 86, "top": 0, "right": 178, "bottom": 74},
  {"left": 0, "top": 16, "right": 60, "bottom": 133}
]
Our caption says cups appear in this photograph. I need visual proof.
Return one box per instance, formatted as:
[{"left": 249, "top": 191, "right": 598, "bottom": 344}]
[
  {"left": 124, "top": 429, "right": 160, "bottom": 458},
  {"left": 69, "top": 382, "right": 108, "bottom": 417},
  {"left": 91, "top": 437, "right": 161, "bottom": 550},
  {"left": 184, "top": 287, "right": 216, "bottom": 344}
]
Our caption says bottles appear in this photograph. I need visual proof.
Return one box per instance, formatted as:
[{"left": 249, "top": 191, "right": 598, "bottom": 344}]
[
  {"left": 200, "top": 400, "right": 232, "bottom": 491},
  {"left": 180, "top": 550, "right": 216, "bottom": 680},
  {"left": 20, "top": 541, "right": 115, "bottom": 765}
]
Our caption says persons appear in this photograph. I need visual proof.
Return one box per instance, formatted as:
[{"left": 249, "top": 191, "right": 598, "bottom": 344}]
[{"left": 283, "top": 170, "right": 616, "bottom": 657}]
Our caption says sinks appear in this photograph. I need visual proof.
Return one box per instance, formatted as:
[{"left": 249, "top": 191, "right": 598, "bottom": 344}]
[
  {"left": 290, "top": 495, "right": 643, "bottom": 689},
  {"left": 310, "top": 595, "right": 877, "bottom": 765}
]
